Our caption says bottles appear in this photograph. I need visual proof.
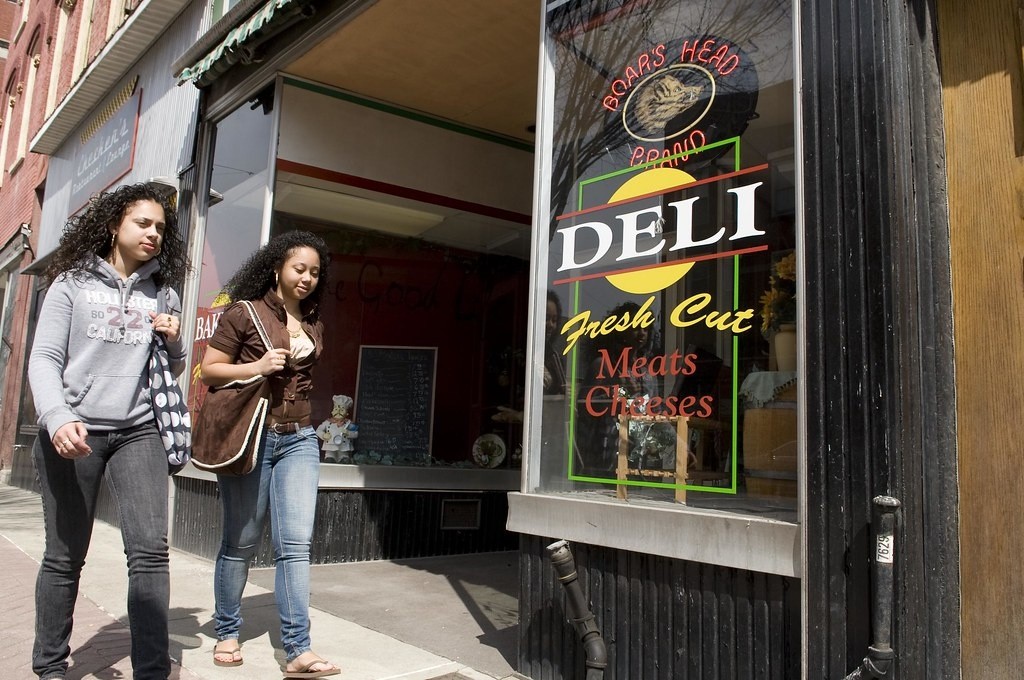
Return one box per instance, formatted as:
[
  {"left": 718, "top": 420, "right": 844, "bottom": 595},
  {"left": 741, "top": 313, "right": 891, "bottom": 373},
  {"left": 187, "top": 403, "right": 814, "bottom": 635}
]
[{"left": 511, "top": 449, "right": 522, "bottom": 470}]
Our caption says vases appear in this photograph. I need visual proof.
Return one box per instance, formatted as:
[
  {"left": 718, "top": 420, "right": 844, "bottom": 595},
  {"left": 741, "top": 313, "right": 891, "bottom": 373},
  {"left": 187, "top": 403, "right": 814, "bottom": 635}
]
[{"left": 766, "top": 318, "right": 798, "bottom": 403}]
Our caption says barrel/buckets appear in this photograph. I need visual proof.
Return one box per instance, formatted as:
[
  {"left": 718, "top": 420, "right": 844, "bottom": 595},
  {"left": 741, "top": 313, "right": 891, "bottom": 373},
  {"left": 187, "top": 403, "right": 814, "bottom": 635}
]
[{"left": 744, "top": 384, "right": 797, "bottom": 502}]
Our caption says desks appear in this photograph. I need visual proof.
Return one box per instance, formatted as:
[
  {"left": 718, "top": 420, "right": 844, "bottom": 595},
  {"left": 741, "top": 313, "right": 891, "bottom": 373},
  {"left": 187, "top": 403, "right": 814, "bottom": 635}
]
[{"left": 617, "top": 411, "right": 740, "bottom": 503}]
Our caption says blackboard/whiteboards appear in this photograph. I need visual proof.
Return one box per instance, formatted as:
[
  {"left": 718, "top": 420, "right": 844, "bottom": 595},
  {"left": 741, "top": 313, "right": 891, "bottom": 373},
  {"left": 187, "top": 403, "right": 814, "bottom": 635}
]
[{"left": 352, "top": 345, "right": 438, "bottom": 466}]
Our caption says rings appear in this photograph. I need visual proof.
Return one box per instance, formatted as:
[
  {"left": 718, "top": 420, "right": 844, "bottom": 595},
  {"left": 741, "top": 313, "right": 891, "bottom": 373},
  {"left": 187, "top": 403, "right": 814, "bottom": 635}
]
[
  {"left": 62, "top": 440, "right": 70, "bottom": 447},
  {"left": 278, "top": 355, "right": 281, "bottom": 360},
  {"left": 167, "top": 316, "right": 171, "bottom": 322},
  {"left": 59, "top": 446, "right": 64, "bottom": 449},
  {"left": 168, "top": 324, "right": 172, "bottom": 328}
]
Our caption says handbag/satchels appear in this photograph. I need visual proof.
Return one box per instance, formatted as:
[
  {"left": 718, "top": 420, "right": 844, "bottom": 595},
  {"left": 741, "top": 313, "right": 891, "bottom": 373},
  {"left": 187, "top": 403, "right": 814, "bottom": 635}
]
[
  {"left": 148, "top": 285, "right": 192, "bottom": 476},
  {"left": 191, "top": 300, "right": 276, "bottom": 477}
]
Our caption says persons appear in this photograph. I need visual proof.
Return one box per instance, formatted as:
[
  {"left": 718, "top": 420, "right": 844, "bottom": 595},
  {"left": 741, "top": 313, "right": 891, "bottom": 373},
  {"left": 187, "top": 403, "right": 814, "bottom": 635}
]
[
  {"left": 315, "top": 393, "right": 359, "bottom": 464},
  {"left": 200, "top": 233, "right": 341, "bottom": 677},
  {"left": 577, "top": 302, "right": 698, "bottom": 495},
  {"left": 541, "top": 288, "right": 583, "bottom": 493},
  {"left": 26, "top": 180, "right": 196, "bottom": 680}
]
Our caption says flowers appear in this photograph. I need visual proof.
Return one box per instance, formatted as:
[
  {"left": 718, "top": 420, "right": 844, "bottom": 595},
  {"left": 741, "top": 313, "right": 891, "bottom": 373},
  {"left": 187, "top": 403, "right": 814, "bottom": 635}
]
[{"left": 758, "top": 248, "right": 798, "bottom": 338}]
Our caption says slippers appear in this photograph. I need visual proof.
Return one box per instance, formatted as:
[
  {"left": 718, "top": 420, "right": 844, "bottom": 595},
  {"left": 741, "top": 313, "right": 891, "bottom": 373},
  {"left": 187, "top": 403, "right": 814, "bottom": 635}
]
[
  {"left": 213, "top": 644, "right": 243, "bottom": 666},
  {"left": 283, "top": 660, "right": 342, "bottom": 678}
]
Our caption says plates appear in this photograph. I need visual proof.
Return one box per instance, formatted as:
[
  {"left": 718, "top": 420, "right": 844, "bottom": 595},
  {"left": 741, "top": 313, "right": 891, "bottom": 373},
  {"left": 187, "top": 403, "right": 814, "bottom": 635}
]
[{"left": 473, "top": 434, "right": 506, "bottom": 469}]
